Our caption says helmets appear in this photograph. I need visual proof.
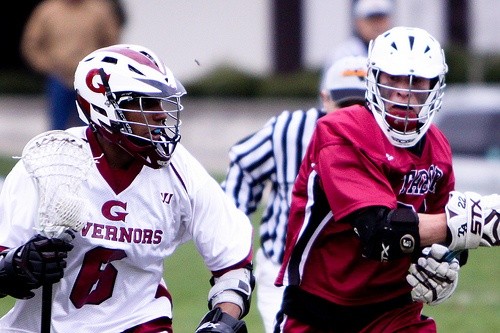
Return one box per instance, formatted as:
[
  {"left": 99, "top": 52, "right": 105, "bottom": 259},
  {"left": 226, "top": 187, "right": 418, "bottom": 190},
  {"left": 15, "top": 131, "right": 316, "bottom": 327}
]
[
  {"left": 73, "top": 44, "right": 187, "bottom": 169},
  {"left": 364, "top": 27, "right": 449, "bottom": 148}
]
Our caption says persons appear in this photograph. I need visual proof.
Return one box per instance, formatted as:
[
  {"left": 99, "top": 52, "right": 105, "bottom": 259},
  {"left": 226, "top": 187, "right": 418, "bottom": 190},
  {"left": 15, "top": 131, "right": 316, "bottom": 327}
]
[
  {"left": 224, "top": 1, "right": 401, "bottom": 333},
  {"left": 276, "top": 27, "right": 500, "bottom": 332},
  {"left": 0, "top": 44, "right": 258, "bottom": 333},
  {"left": 22, "top": 0, "right": 126, "bottom": 134}
]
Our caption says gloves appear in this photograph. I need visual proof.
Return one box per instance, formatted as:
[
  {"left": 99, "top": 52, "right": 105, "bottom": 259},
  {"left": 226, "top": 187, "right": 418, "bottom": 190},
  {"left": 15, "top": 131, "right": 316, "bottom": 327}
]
[
  {"left": 405, "top": 245, "right": 460, "bottom": 306},
  {"left": 445, "top": 191, "right": 500, "bottom": 252},
  {"left": 194, "top": 307, "right": 248, "bottom": 333},
  {"left": 10, "top": 236, "right": 74, "bottom": 287}
]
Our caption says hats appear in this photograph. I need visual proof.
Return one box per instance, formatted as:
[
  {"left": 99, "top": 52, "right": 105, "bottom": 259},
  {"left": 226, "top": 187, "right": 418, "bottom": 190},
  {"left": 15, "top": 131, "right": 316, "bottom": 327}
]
[
  {"left": 352, "top": 0, "right": 395, "bottom": 19},
  {"left": 323, "top": 56, "right": 368, "bottom": 103}
]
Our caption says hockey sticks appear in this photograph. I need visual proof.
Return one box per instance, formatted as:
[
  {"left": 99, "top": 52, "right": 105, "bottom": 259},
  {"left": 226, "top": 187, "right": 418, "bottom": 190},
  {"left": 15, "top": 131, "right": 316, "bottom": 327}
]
[
  {"left": 442, "top": 248, "right": 462, "bottom": 264},
  {"left": 41, "top": 282, "right": 54, "bottom": 333}
]
